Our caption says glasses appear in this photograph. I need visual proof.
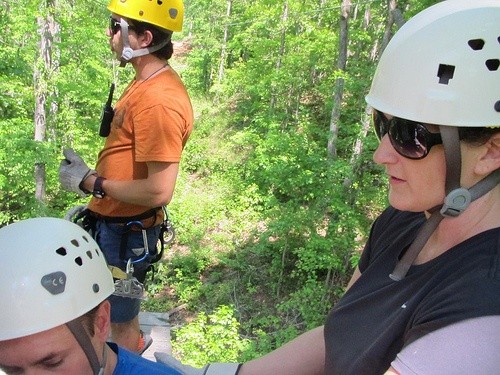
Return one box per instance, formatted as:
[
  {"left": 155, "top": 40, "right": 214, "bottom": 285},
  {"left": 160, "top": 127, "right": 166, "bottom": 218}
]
[{"left": 372, "top": 109, "right": 493, "bottom": 160}]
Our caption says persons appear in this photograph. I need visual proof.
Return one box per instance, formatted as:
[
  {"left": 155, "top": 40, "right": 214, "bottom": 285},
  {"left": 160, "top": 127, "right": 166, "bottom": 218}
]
[
  {"left": 154, "top": 0, "right": 500, "bottom": 375},
  {"left": 59, "top": 1, "right": 194, "bottom": 356},
  {"left": 0, "top": 216, "right": 175, "bottom": 374}
]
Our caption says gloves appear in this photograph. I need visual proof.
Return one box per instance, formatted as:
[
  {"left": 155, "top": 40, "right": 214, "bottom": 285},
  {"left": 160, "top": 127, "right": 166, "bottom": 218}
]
[
  {"left": 66, "top": 203, "right": 87, "bottom": 220},
  {"left": 59, "top": 149, "right": 98, "bottom": 196},
  {"left": 155, "top": 352, "right": 242, "bottom": 375}
]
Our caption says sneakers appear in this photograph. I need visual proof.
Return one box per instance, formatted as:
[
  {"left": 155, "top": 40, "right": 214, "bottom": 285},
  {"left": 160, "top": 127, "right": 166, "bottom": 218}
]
[{"left": 136, "top": 330, "right": 153, "bottom": 356}]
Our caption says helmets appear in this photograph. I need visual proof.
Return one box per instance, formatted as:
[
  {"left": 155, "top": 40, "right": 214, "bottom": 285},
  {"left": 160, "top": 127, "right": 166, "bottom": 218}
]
[
  {"left": 365, "top": 0, "right": 500, "bottom": 127},
  {"left": 106, "top": 0, "right": 183, "bottom": 32},
  {"left": 0, "top": 217, "right": 115, "bottom": 340}
]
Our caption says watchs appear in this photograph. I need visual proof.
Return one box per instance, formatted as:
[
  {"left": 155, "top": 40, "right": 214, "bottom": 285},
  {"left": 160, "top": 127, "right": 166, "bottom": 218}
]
[{"left": 92, "top": 177, "right": 107, "bottom": 200}]
